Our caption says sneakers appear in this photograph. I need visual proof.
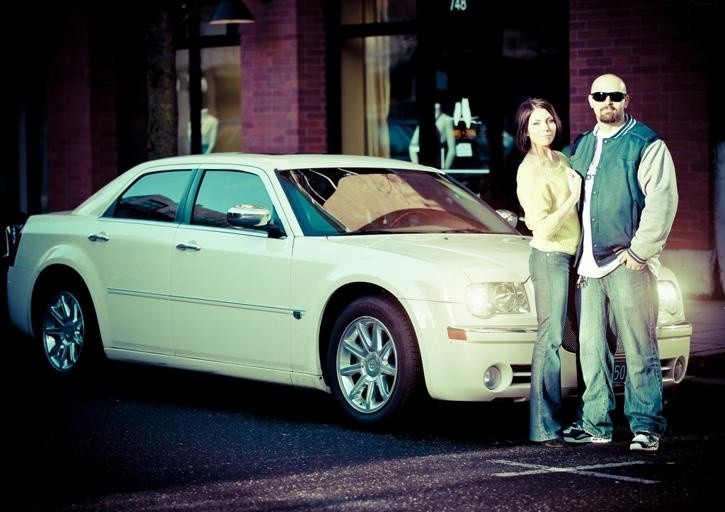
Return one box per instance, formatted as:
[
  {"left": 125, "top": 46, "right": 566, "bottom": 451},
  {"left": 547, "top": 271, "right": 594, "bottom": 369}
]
[
  {"left": 562, "top": 420, "right": 614, "bottom": 444},
  {"left": 629, "top": 432, "right": 664, "bottom": 451}
]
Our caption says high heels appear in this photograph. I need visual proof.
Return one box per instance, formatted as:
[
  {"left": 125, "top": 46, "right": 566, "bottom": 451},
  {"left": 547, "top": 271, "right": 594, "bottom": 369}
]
[{"left": 545, "top": 436, "right": 569, "bottom": 448}]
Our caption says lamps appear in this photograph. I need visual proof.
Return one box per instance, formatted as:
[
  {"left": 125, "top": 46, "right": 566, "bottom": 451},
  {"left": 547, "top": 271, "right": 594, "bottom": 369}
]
[{"left": 210, "top": 0, "right": 256, "bottom": 25}]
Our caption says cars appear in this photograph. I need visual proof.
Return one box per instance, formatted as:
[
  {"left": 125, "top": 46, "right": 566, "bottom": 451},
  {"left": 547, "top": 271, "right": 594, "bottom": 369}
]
[{"left": 3, "top": 152, "right": 693, "bottom": 433}]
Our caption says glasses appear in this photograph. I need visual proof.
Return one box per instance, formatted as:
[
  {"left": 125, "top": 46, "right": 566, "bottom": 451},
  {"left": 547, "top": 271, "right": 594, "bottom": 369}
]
[{"left": 592, "top": 92, "right": 627, "bottom": 102}]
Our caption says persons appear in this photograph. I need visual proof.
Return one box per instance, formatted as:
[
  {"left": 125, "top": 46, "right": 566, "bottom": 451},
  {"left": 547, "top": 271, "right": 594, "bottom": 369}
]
[
  {"left": 560, "top": 74, "right": 682, "bottom": 453},
  {"left": 511, "top": 94, "right": 585, "bottom": 448}
]
